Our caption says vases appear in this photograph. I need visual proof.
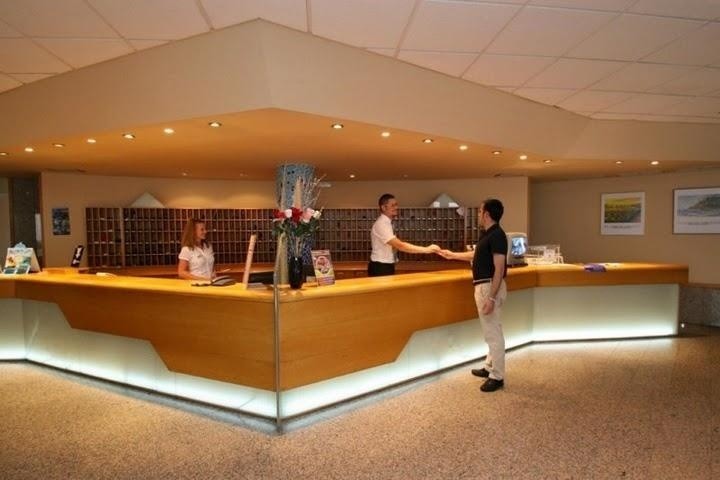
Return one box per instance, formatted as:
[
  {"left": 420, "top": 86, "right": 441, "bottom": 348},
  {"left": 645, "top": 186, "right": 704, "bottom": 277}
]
[{"left": 288, "top": 256, "right": 304, "bottom": 288}]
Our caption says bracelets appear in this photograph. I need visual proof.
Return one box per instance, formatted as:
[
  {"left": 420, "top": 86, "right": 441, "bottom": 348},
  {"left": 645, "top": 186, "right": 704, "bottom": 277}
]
[{"left": 488, "top": 295, "right": 496, "bottom": 303}]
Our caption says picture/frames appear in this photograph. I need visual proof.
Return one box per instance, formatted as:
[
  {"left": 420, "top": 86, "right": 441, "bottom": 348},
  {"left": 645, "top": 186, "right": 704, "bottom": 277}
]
[
  {"left": 600, "top": 191, "right": 645, "bottom": 237},
  {"left": 672, "top": 186, "right": 720, "bottom": 235}
]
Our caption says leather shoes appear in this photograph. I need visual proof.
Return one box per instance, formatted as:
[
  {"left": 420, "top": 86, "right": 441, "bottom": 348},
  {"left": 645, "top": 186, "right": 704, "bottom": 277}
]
[{"left": 472, "top": 369, "right": 504, "bottom": 392}]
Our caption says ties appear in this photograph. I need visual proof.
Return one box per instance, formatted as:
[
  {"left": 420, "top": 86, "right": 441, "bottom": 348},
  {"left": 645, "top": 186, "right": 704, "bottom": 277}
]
[{"left": 391, "top": 221, "right": 399, "bottom": 262}]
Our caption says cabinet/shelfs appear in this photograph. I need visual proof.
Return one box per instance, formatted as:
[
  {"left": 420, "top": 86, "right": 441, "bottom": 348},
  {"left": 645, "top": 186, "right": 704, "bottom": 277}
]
[{"left": 84, "top": 207, "right": 484, "bottom": 267}]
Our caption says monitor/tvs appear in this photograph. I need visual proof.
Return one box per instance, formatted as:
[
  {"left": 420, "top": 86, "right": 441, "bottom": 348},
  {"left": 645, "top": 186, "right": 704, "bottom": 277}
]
[{"left": 249, "top": 271, "right": 274, "bottom": 284}]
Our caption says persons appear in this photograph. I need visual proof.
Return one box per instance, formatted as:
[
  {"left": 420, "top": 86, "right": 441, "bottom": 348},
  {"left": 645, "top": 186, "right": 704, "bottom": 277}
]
[
  {"left": 368, "top": 193, "right": 441, "bottom": 277},
  {"left": 438, "top": 199, "right": 508, "bottom": 392},
  {"left": 176, "top": 217, "right": 217, "bottom": 280}
]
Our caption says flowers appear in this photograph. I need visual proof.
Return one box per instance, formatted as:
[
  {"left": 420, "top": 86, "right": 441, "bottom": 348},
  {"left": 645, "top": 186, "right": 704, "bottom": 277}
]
[{"left": 272, "top": 205, "right": 322, "bottom": 257}]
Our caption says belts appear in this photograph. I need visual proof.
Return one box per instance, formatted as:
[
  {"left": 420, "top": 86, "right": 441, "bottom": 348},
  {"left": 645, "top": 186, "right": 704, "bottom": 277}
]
[{"left": 474, "top": 279, "right": 490, "bottom": 285}]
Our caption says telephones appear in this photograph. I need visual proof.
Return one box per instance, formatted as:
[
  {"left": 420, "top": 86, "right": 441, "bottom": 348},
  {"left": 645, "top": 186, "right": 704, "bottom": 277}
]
[{"left": 211, "top": 276, "right": 235, "bottom": 286}]
